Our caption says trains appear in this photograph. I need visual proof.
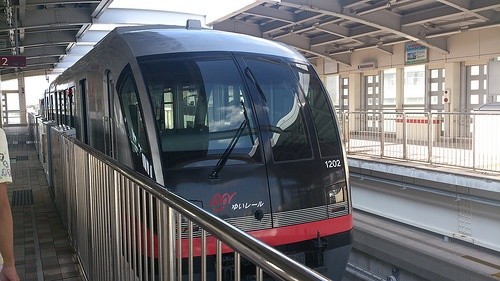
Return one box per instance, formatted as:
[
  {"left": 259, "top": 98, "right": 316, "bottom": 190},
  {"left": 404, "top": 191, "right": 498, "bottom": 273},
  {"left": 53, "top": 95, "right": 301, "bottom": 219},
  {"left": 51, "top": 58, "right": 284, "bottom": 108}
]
[{"left": 35, "top": 19, "right": 355, "bottom": 281}]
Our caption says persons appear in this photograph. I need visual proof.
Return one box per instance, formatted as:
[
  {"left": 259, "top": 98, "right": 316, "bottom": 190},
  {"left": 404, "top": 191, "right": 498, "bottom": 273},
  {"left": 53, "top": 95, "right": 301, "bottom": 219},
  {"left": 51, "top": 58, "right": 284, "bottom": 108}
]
[{"left": 0, "top": 127, "right": 22, "bottom": 281}]
